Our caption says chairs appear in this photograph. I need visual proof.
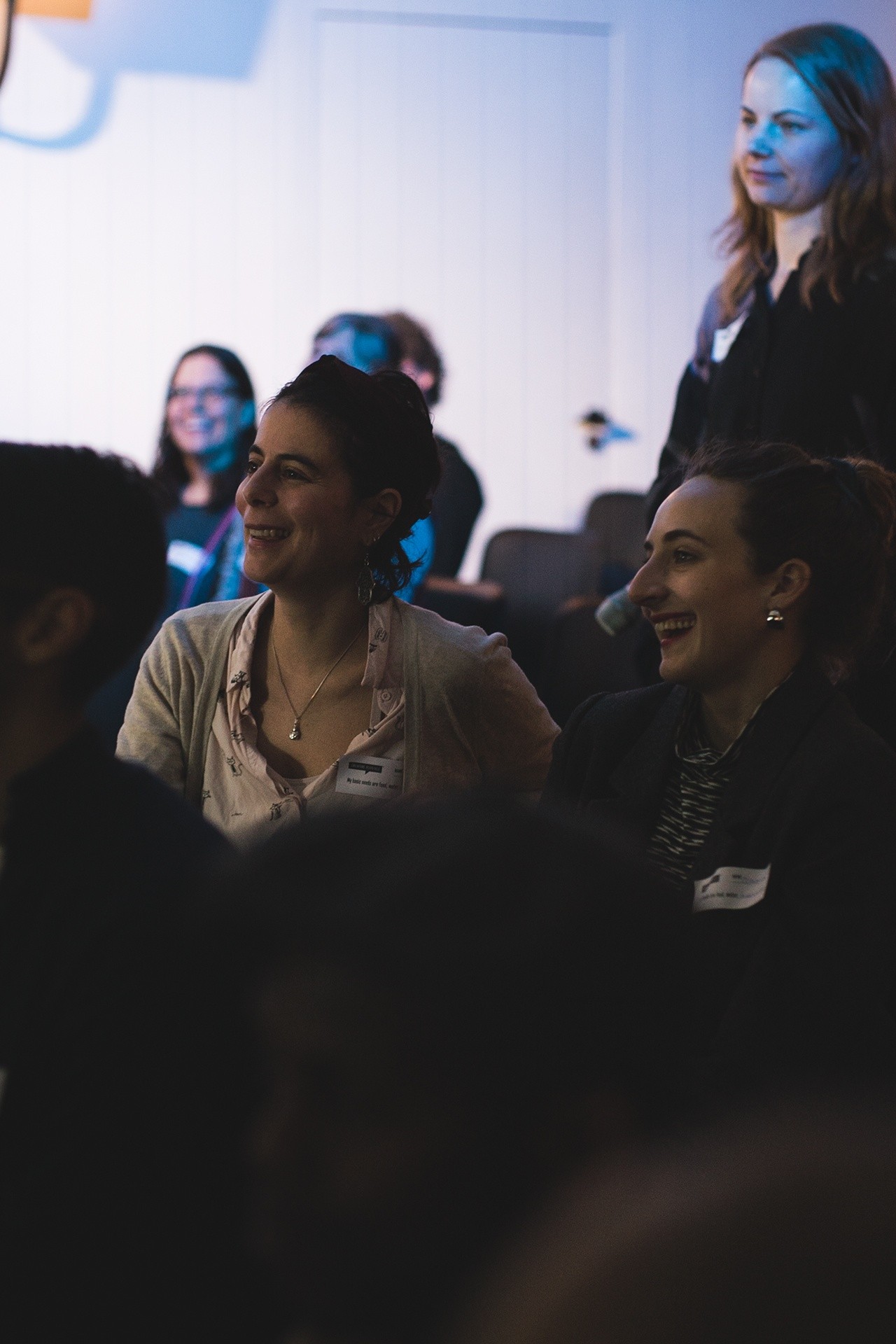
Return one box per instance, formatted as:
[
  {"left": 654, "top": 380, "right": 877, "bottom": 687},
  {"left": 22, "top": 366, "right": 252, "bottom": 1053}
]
[
  {"left": 538, "top": 596, "right": 645, "bottom": 723},
  {"left": 479, "top": 530, "right": 604, "bottom": 665},
  {"left": 583, "top": 491, "right": 650, "bottom": 573},
  {"left": 419, "top": 579, "right": 505, "bottom": 635}
]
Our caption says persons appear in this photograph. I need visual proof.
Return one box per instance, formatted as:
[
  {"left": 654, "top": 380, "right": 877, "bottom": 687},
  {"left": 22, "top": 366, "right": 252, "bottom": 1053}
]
[
  {"left": 583, "top": 409, "right": 631, "bottom": 450},
  {"left": 215, "top": 312, "right": 433, "bottom": 601},
  {"left": 176, "top": 787, "right": 713, "bottom": 1344},
  {"left": 385, "top": 310, "right": 484, "bottom": 579},
  {"left": 0, "top": 440, "right": 256, "bottom": 1344},
  {"left": 546, "top": 434, "right": 896, "bottom": 935},
  {"left": 146, "top": 345, "right": 258, "bottom": 612},
  {"left": 472, "top": 1101, "right": 896, "bottom": 1344},
  {"left": 114, "top": 354, "right": 561, "bottom": 849},
  {"left": 655, "top": 24, "right": 896, "bottom": 459}
]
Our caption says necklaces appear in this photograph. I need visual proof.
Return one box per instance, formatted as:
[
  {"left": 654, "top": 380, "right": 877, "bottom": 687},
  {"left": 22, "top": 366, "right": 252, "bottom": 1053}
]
[{"left": 270, "top": 621, "right": 367, "bottom": 739}]
